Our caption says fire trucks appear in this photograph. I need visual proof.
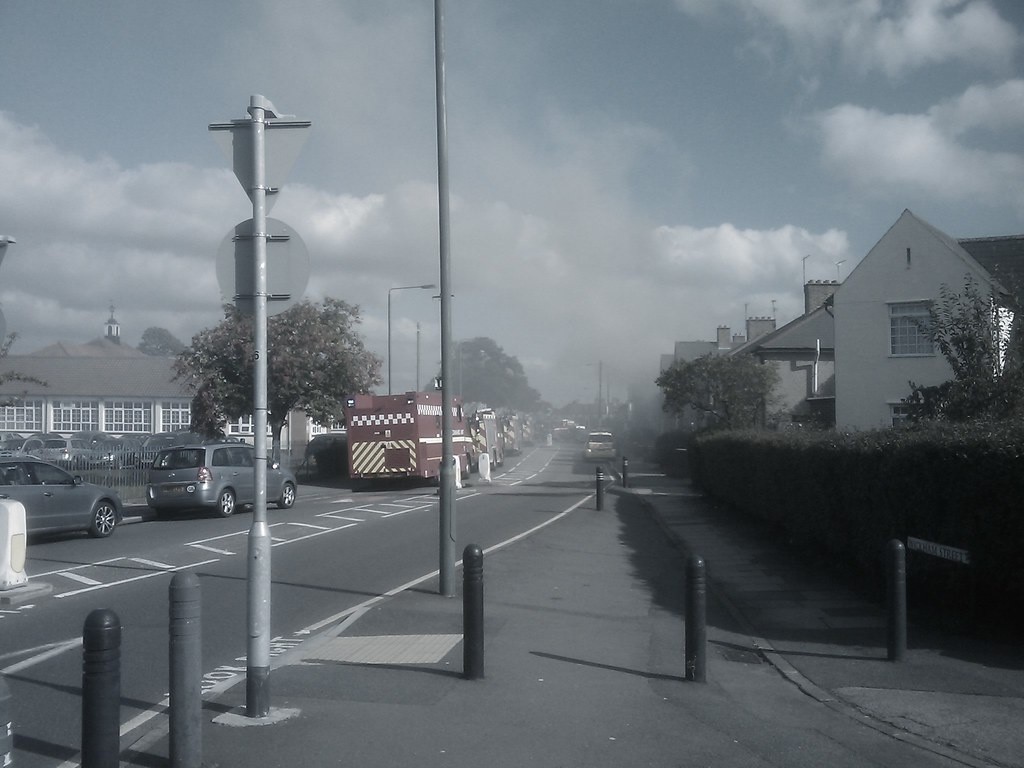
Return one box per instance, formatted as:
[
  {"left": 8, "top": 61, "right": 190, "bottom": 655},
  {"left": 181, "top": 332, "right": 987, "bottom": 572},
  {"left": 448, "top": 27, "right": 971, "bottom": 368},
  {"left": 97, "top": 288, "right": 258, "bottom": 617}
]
[{"left": 344, "top": 391, "right": 535, "bottom": 490}]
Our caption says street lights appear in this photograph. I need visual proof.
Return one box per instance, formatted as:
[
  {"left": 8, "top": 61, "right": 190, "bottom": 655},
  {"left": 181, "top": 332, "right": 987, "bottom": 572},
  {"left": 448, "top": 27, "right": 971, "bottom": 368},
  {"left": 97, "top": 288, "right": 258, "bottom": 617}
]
[{"left": 387, "top": 282, "right": 439, "bottom": 394}]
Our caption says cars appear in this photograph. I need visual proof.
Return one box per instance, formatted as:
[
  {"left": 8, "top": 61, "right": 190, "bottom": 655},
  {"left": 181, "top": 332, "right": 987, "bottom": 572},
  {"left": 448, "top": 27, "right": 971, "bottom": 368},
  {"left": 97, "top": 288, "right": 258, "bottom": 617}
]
[
  {"left": 584, "top": 429, "right": 618, "bottom": 463},
  {"left": 147, "top": 439, "right": 298, "bottom": 519},
  {"left": 0, "top": 454, "right": 125, "bottom": 541},
  {"left": 0, "top": 429, "right": 228, "bottom": 451},
  {"left": 551, "top": 418, "right": 588, "bottom": 440}
]
